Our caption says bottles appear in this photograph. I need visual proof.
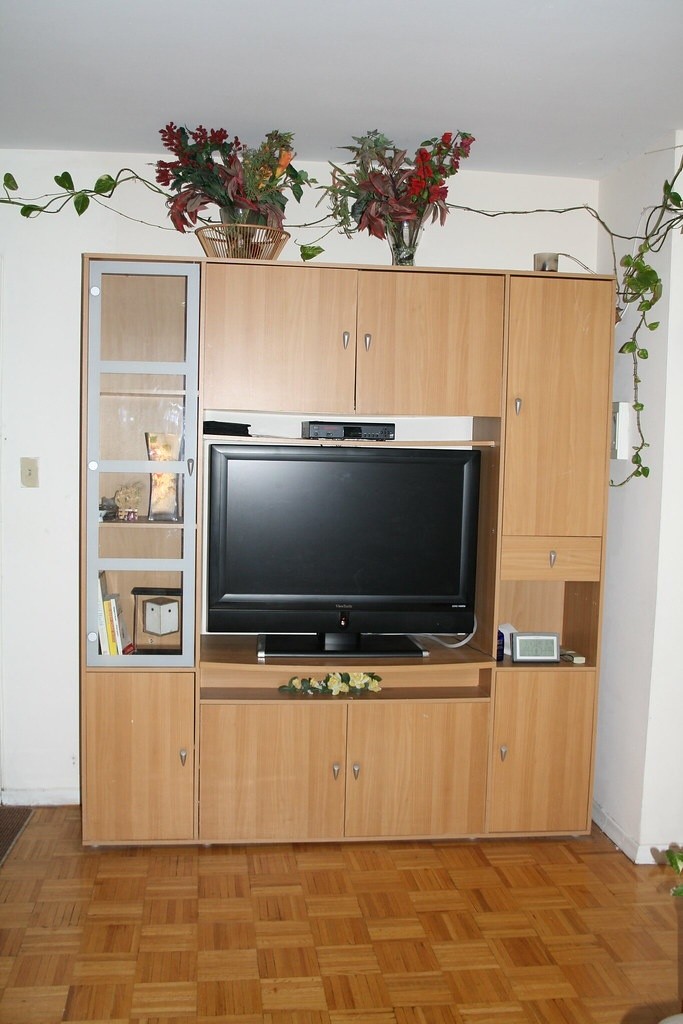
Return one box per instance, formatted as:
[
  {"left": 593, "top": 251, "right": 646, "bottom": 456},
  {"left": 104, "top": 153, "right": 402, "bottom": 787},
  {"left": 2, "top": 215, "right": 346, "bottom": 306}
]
[{"left": 497, "top": 629, "right": 504, "bottom": 661}]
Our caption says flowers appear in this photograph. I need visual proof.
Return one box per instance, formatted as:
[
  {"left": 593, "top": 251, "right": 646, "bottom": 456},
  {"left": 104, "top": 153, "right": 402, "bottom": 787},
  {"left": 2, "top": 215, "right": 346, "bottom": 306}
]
[
  {"left": 317, "top": 127, "right": 476, "bottom": 239},
  {"left": 279, "top": 672, "right": 383, "bottom": 700},
  {"left": 150, "top": 117, "right": 314, "bottom": 232}
]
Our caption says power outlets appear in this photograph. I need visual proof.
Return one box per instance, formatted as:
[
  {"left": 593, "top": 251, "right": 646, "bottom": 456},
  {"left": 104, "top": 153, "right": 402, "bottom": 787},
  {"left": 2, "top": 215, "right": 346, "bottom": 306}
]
[{"left": 534, "top": 252, "right": 561, "bottom": 271}]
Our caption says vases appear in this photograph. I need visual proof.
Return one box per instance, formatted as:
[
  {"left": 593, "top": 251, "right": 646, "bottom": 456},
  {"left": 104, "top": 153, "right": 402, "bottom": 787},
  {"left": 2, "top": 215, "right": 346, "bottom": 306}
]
[
  {"left": 194, "top": 225, "right": 290, "bottom": 261},
  {"left": 382, "top": 219, "right": 421, "bottom": 268}
]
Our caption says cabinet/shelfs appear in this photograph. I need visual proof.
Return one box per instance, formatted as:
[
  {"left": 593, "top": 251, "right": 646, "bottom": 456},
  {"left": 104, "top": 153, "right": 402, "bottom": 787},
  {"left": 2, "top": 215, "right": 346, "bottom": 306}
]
[{"left": 79, "top": 252, "right": 618, "bottom": 846}]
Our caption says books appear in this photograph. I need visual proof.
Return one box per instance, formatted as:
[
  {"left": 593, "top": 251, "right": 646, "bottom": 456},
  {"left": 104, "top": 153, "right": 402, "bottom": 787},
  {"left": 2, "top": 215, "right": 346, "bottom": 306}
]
[
  {"left": 104, "top": 594, "right": 118, "bottom": 656},
  {"left": 111, "top": 593, "right": 135, "bottom": 655},
  {"left": 98, "top": 569, "right": 110, "bottom": 657}
]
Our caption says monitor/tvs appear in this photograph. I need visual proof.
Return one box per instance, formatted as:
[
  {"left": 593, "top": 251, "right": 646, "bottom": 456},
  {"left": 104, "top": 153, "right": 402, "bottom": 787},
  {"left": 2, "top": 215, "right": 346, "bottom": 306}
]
[{"left": 207, "top": 447, "right": 481, "bottom": 657}]
